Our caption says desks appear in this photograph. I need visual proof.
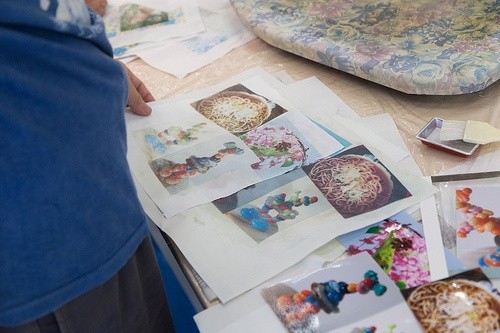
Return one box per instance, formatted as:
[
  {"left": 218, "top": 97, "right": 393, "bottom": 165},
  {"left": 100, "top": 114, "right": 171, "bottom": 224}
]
[{"left": 118, "top": 34, "right": 500, "bottom": 333}]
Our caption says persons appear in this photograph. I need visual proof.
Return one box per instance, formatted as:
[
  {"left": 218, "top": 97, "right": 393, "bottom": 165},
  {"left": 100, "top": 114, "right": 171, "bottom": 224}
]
[{"left": 1, "top": 0, "right": 177, "bottom": 333}]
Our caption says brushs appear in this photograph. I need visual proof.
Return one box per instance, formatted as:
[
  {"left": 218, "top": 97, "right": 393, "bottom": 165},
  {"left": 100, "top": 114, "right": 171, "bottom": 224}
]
[{"left": 441, "top": 119, "right": 500, "bottom": 146}]
